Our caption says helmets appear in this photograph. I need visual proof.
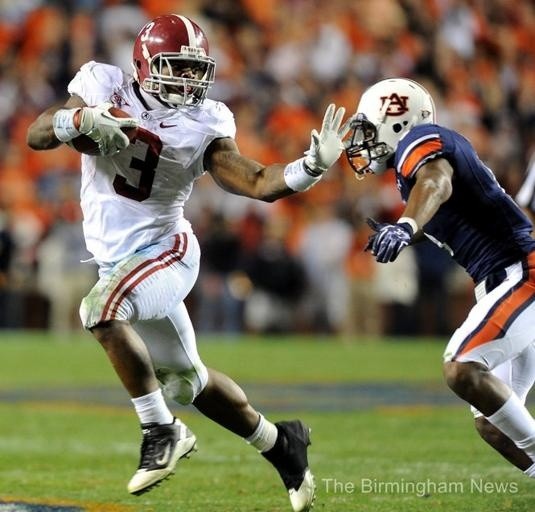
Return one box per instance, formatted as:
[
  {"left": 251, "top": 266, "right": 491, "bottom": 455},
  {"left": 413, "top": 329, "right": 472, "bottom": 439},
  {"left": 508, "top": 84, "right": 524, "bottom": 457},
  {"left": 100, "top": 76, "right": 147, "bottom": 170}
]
[
  {"left": 345, "top": 76, "right": 437, "bottom": 180},
  {"left": 132, "top": 15, "right": 217, "bottom": 114}
]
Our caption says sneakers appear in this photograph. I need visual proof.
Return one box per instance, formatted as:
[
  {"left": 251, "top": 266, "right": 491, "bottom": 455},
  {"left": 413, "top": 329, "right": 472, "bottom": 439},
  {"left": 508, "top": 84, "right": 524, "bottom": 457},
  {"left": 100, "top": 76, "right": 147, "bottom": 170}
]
[
  {"left": 128, "top": 419, "right": 198, "bottom": 495},
  {"left": 259, "top": 419, "right": 317, "bottom": 512}
]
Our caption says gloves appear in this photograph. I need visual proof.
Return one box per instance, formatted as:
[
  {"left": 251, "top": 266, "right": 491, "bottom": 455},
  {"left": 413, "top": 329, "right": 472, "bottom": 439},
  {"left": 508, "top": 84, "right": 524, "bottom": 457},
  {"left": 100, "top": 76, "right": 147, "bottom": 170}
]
[
  {"left": 365, "top": 217, "right": 415, "bottom": 264},
  {"left": 305, "top": 101, "right": 353, "bottom": 174},
  {"left": 78, "top": 103, "right": 139, "bottom": 158}
]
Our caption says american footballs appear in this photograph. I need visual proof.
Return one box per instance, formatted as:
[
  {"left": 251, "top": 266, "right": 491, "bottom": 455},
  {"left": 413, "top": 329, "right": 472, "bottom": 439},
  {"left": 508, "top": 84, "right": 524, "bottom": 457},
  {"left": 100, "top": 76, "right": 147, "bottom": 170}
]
[{"left": 64, "top": 106, "right": 138, "bottom": 157}]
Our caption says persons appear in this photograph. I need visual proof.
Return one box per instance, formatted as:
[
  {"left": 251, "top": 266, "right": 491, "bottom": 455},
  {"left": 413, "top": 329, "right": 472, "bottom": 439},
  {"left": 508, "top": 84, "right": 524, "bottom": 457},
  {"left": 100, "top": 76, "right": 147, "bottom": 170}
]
[
  {"left": 22, "top": 8, "right": 362, "bottom": 510},
  {"left": 1, "top": 1, "right": 535, "bottom": 335},
  {"left": 334, "top": 75, "right": 535, "bottom": 481}
]
